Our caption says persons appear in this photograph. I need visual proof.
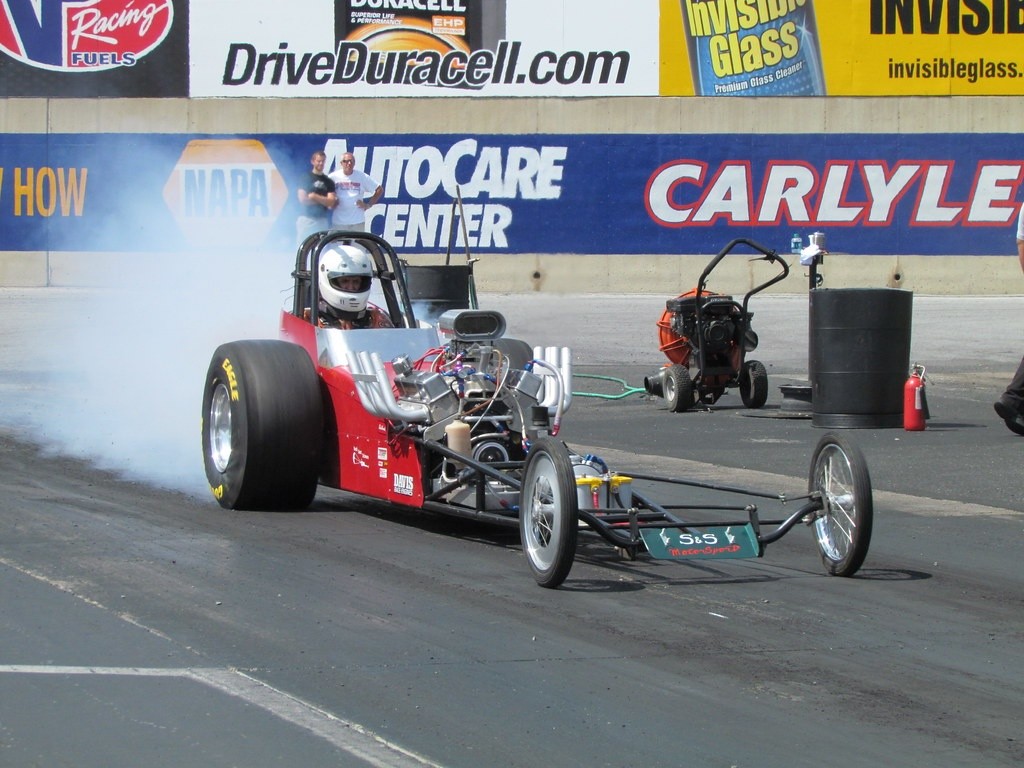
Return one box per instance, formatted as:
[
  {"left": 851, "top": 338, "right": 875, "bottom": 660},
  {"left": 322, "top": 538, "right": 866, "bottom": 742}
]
[
  {"left": 295, "top": 151, "right": 337, "bottom": 246},
  {"left": 994, "top": 198, "right": 1024, "bottom": 435},
  {"left": 295, "top": 243, "right": 392, "bottom": 329},
  {"left": 326, "top": 152, "right": 383, "bottom": 233}
]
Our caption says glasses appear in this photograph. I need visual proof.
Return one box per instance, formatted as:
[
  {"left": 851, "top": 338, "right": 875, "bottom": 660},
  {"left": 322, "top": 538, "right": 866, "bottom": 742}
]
[{"left": 341, "top": 160, "right": 355, "bottom": 163}]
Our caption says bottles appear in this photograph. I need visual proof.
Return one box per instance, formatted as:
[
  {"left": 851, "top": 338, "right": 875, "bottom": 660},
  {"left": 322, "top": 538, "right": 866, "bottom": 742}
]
[
  {"left": 791, "top": 234, "right": 802, "bottom": 253},
  {"left": 445, "top": 418, "right": 473, "bottom": 471}
]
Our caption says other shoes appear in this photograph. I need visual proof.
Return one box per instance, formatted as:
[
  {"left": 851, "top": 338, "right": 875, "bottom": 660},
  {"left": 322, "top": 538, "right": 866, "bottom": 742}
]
[{"left": 994, "top": 399, "right": 1024, "bottom": 436}]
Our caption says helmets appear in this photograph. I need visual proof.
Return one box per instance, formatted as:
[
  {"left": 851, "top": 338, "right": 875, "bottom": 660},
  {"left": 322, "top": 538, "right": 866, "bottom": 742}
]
[{"left": 317, "top": 238, "right": 373, "bottom": 319}]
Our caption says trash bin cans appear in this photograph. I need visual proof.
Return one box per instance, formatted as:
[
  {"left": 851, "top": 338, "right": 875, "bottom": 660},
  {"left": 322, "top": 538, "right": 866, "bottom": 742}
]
[
  {"left": 809, "top": 286, "right": 915, "bottom": 430},
  {"left": 400, "top": 259, "right": 470, "bottom": 331}
]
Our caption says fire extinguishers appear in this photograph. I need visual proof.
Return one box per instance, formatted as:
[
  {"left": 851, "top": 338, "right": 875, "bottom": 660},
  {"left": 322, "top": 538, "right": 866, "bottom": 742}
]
[{"left": 903, "top": 362, "right": 931, "bottom": 431}]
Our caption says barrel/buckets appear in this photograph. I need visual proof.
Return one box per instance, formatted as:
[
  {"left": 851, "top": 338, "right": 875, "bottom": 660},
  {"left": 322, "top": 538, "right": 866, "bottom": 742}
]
[
  {"left": 809, "top": 289, "right": 914, "bottom": 428},
  {"left": 403, "top": 265, "right": 472, "bottom": 327}
]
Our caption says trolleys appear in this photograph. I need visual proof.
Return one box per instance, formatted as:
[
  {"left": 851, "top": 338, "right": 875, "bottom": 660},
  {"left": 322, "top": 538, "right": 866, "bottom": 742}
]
[{"left": 643, "top": 238, "right": 790, "bottom": 412}]
